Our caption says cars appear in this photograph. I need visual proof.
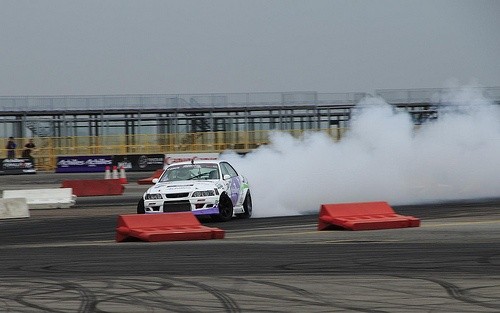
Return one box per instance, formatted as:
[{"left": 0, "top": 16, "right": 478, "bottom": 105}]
[
  {"left": 133, "top": 159, "right": 165, "bottom": 172},
  {"left": 136, "top": 161, "right": 253, "bottom": 222}
]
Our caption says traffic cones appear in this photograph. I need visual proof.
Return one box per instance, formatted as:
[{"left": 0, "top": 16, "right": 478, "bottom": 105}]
[
  {"left": 112, "top": 166, "right": 119, "bottom": 179},
  {"left": 103, "top": 166, "right": 112, "bottom": 179},
  {"left": 119, "top": 166, "right": 129, "bottom": 184}
]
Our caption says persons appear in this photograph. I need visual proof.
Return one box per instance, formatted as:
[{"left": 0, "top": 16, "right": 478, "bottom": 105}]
[
  {"left": 21, "top": 139, "right": 36, "bottom": 169},
  {"left": 5, "top": 136, "right": 16, "bottom": 160}
]
